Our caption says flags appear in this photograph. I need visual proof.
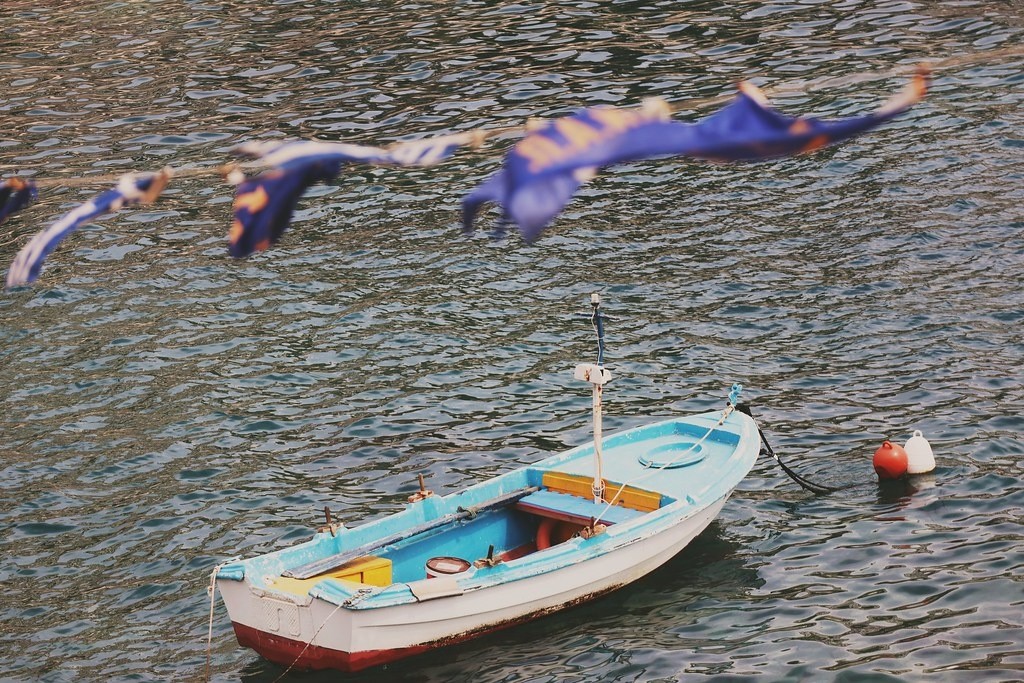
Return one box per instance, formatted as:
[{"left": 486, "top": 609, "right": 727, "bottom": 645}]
[
  {"left": 463, "top": 59, "right": 933, "bottom": 246},
  {"left": 5, "top": 168, "right": 170, "bottom": 288},
  {"left": 219, "top": 127, "right": 484, "bottom": 259}
]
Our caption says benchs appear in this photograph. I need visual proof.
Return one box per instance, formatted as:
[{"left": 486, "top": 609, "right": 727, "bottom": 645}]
[{"left": 504, "top": 488, "right": 649, "bottom": 526}]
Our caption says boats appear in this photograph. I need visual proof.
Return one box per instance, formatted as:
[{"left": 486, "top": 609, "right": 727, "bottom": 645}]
[{"left": 209, "top": 293, "right": 768, "bottom": 680}]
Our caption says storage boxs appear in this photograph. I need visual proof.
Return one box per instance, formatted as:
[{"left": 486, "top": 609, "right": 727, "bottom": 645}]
[
  {"left": 273, "top": 555, "right": 392, "bottom": 595},
  {"left": 424, "top": 556, "right": 471, "bottom": 578}
]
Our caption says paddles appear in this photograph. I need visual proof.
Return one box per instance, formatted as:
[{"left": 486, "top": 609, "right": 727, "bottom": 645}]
[{"left": 280, "top": 485, "right": 541, "bottom": 578}]
